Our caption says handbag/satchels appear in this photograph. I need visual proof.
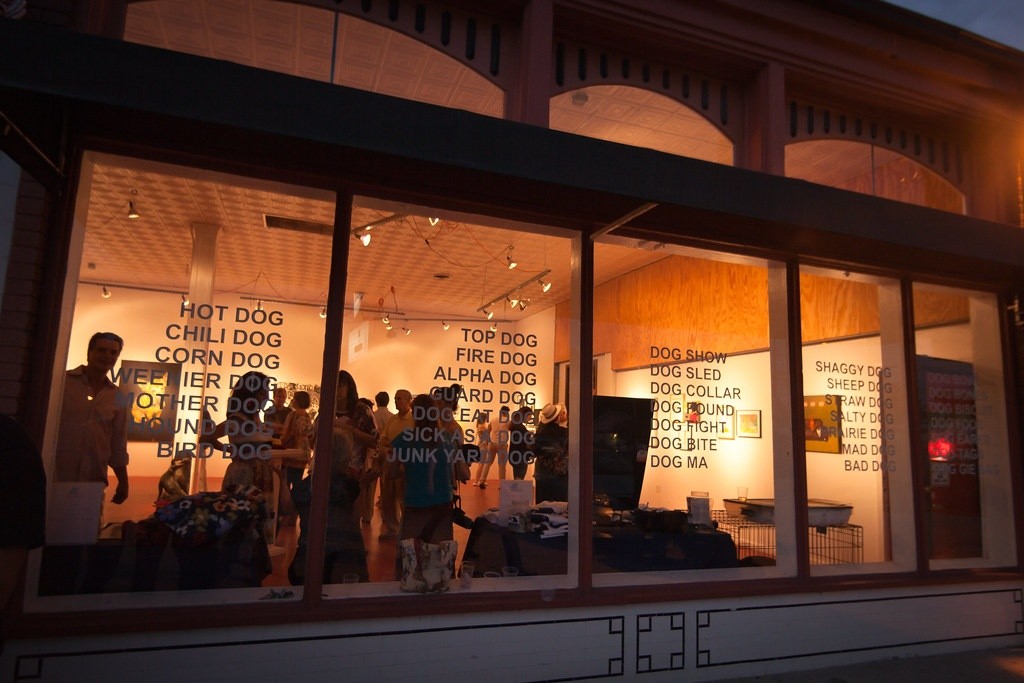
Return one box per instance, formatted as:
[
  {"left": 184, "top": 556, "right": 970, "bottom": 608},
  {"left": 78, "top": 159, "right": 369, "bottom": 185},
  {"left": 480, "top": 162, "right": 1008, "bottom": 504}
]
[{"left": 399, "top": 537, "right": 457, "bottom": 593}]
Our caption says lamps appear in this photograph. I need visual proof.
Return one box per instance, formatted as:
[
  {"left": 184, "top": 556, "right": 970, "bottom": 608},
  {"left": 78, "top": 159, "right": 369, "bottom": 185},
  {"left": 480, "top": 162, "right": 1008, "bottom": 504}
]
[
  {"left": 101, "top": 285, "right": 112, "bottom": 299},
  {"left": 255, "top": 300, "right": 264, "bottom": 310},
  {"left": 385, "top": 323, "right": 393, "bottom": 331},
  {"left": 354, "top": 232, "right": 371, "bottom": 247},
  {"left": 401, "top": 321, "right": 411, "bottom": 335},
  {"left": 441, "top": 321, "right": 450, "bottom": 330},
  {"left": 181, "top": 294, "right": 189, "bottom": 307},
  {"left": 506, "top": 296, "right": 519, "bottom": 309},
  {"left": 382, "top": 313, "right": 390, "bottom": 323},
  {"left": 538, "top": 279, "right": 552, "bottom": 293},
  {"left": 483, "top": 308, "right": 493, "bottom": 320},
  {"left": 429, "top": 217, "right": 440, "bottom": 226},
  {"left": 506, "top": 256, "right": 518, "bottom": 271},
  {"left": 319, "top": 308, "right": 327, "bottom": 319},
  {"left": 127, "top": 200, "right": 140, "bottom": 219},
  {"left": 519, "top": 295, "right": 530, "bottom": 311},
  {"left": 490, "top": 322, "right": 498, "bottom": 332}
]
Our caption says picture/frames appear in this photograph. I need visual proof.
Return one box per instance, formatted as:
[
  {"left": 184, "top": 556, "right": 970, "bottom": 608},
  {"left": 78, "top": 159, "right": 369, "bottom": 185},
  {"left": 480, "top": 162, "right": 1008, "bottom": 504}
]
[
  {"left": 119, "top": 360, "right": 182, "bottom": 444},
  {"left": 683, "top": 401, "right": 700, "bottom": 423},
  {"left": 804, "top": 395, "right": 842, "bottom": 454},
  {"left": 716, "top": 414, "right": 735, "bottom": 440},
  {"left": 736, "top": 410, "right": 762, "bottom": 438},
  {"left": 670, "top": 393, "right": 686, "bottom": 424}
]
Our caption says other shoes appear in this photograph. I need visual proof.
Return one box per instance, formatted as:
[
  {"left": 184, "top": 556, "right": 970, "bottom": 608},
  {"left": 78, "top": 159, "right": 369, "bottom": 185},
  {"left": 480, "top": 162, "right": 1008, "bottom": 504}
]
[
  {"left": 378, "top": 535, "right": 397, "bottom": 540},
  {"left": 362, "top": 519, "right": 371, "bottom": 524},
  {"left": 267, "top": 543, "right": 287, "bottom": 557},
  {"left": 473, "top": 481, "right": 488, "bottom": 489}
]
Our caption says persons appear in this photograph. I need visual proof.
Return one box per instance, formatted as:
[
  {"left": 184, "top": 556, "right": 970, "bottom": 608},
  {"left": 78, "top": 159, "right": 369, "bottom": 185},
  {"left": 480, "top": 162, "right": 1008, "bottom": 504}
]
[
  {"left": 199, "top": 371, "right": 313, "bottom": 524},
  {"left": 0, "top": 412, "right": 47, "bottom": 610},
  {"left": 52, "top": 332, "right": 130, "bottom": 503},
  {"left": 472, "top": 407, "right": 532, "bottom": 489},
  {"left": 290, "top": 370, "right": 381, "bottom": 583},
  {"left": 528, "top": 403, "right": 569, "bottom": 505},
  {"left": 359, "top": 384, "right": 464, "bottom": 580}
]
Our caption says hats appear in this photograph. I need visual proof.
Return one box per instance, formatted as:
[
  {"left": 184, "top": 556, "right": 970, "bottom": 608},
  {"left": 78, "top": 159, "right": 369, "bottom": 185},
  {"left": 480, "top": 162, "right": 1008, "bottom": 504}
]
[{"left": 542, "top": 403, "right": 562, "bottom": 424}]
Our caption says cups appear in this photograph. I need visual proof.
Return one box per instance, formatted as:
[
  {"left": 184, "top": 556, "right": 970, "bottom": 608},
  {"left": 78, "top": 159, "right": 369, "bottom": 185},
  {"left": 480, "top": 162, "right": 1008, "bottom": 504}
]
[
  {"left": 460, "top": 561, "right": 475, "bottom": 589},
  {"left": 502, "top": 566, "right": 519, "bottom": 591},
  {"left": 737, "top": 486, "right": 748, "bottom": 503},
  {"left": 343, "top": 573, "right": 360, "bottom": 595},
  {"left": 483, "top": 572, "right": 501, "bottom": 592}
]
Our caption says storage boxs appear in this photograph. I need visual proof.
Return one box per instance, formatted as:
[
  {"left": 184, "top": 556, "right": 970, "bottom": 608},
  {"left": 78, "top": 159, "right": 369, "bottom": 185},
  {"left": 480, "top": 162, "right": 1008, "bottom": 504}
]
[
  {"left": 722, "top": 497, "right": 854, "bottom": 528},
  {"left": 675, "top": 509, "right": 865, "bottom": 566},
  {"left": 500, "top": 478, "right": 535, "bottom": 527}
]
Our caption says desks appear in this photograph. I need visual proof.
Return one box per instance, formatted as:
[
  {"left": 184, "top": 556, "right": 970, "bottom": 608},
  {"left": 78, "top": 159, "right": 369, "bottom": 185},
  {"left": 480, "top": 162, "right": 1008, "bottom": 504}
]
[{"left": 454, "top": 516, "right": 738, "bottom": 578}]
